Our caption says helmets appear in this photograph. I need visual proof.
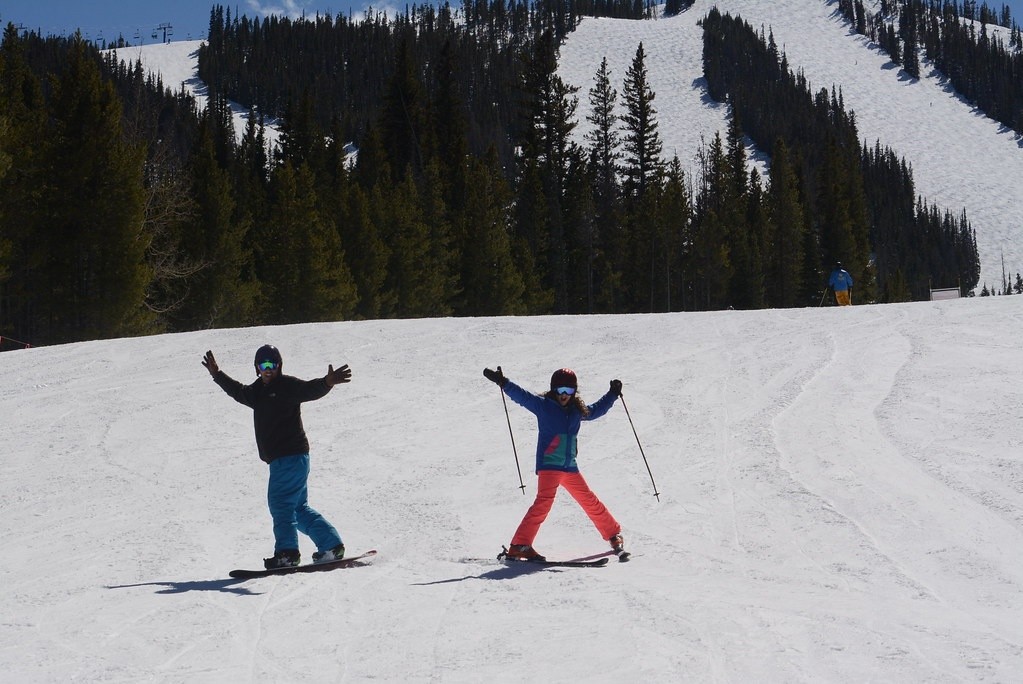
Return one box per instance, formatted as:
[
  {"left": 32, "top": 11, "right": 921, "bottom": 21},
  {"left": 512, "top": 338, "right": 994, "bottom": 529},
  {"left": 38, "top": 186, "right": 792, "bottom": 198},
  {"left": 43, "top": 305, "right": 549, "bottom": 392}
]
[
  {"left": 550, "top": 368, "right": 576, "bottom": 387},
  {"left": 254, "top": 345, "right": 281, "bottom": 372},
  {"left": 836, "top": 261, "right": 841, "bottom": 266}
]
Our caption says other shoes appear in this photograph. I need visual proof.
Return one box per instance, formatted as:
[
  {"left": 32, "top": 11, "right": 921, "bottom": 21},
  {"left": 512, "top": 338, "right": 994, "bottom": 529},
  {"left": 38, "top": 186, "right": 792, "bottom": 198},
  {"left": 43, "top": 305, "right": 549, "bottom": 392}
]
[
  {"left": 264, "top": 549, "right": 300, "bottom": 569},
  {"left": 311, "top": 545, "right": 344, "bottom": 563}
]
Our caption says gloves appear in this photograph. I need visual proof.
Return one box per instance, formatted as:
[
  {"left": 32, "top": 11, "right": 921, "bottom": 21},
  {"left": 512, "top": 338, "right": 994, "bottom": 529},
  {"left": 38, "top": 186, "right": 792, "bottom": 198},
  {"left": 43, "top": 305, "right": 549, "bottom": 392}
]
[
  {"left": 609, "top": 379, "right": 622, "bottom": 395},
  {"left": 482, "top": 366, "right": 506, "bottom": 385}
]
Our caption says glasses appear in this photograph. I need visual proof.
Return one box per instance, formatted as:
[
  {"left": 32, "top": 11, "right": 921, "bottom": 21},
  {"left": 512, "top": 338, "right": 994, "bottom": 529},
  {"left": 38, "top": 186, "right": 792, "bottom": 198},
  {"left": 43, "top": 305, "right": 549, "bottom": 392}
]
[
  {"left": 553, "top": 386, "right": 575, "bottom": 395},
  {"left": 258, "top": 362, "right": 277, "bottom": 371}
]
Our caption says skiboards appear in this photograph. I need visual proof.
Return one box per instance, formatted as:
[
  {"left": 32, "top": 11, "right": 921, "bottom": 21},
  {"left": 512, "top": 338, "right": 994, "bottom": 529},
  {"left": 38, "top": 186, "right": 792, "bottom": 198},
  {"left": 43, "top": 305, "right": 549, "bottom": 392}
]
[{"left": 504, "top": 544, "right": 632, "bottom": 568}]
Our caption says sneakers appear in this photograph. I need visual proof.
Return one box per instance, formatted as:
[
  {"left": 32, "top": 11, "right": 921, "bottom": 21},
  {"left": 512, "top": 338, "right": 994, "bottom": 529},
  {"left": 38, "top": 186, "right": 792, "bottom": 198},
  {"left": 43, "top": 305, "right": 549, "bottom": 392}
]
[
  {"left": 507, "top": 544, "right": 545, "bottom": 562},
  {"left": 609, "top": 533, "right": 624, "bottom": 550}
]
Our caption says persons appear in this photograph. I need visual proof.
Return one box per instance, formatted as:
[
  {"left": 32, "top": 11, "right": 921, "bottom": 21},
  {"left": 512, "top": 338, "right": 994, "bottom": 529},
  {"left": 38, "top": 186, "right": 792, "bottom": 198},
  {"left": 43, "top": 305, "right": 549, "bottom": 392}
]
[
  {"left": 484, "top": 366, "right": 631, "bottom": 562},
  {"left": 202, "top": 344, "right": 352, "bottom": 570},
  {"left": 828, "top": 262, "right": 853, "bottom": 305}
]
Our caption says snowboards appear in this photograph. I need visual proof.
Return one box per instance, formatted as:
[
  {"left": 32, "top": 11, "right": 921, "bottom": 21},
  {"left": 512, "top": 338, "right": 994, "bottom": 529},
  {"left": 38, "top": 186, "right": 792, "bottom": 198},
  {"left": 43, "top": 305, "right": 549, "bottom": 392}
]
[{"left": 229, "top": 549, "right": 378, "bottom": 578}]
